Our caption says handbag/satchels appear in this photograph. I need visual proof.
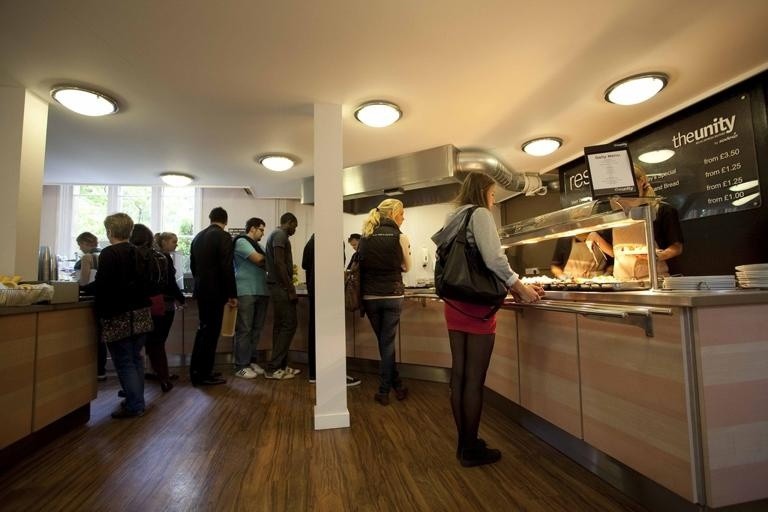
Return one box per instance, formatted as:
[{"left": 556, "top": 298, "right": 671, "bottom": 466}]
[
  {"left": 344, "top": 251, "right": 362, "bottom": 311},
  {"left": 435, "top": 205, "right": 508, "bottom": 305},
  {"left": 149, "top": 293, "right": 167, "bottom": 317}
]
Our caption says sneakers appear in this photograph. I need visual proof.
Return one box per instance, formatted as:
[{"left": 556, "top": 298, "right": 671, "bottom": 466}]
[
  {"left": 456, "top": 439, "right": 485, "bottom": 460},
  {"left": 346, "top": 376, "right": 360, "bottom": 386},
  {"left": 396, "top": 387, "right": 408, "bottom": 401},
  {"left": 264, "top": 367, "right": 294, "bottom": 380},
  {"left": 231, "top": 367, "right": 256, "bottom": 378},
  {"left": 250, "top": 363, "right": 264, "bottom": 375},
  {"left": 460, "top": 446, "right": 500, "bottom": 467},
  {"left": 374, "top": 393, "right": 389, "bottom": 406},
  {"left": 283, "top": 367, "right": 301, "bottom": 375}
]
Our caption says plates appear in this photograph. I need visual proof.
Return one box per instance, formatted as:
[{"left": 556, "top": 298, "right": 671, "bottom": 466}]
[
  {"left": 735, "top": 263, "right": 768, "bottom": 289},
  {"left": 663, "top": 274, "right": 735, "bottom": 290}
]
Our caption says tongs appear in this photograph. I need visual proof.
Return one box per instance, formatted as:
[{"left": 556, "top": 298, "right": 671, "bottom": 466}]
[{"left": 591, "top": 241, "right": 607, "bottom": 265}]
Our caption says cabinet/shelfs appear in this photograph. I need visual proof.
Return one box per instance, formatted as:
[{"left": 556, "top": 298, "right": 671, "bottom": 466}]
[
  {"left": 101, "top": 294, "right": 767, "bottom": 509},
  {"left": 0, "top": 302, "right": 98, "bottom": 468}
]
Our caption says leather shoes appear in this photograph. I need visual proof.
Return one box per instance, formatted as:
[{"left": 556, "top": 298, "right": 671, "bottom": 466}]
[
  {"left": 111, "top": 408, "right": 143, "bottom": 418},
  {"left": 118, "top": 389, "right": 126, "bottom": 396},
  {"left": 200, "top": 376, "right": 225, "bottom": 385}
]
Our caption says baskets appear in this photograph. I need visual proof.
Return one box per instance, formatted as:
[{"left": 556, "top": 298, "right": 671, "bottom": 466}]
[{"left": 0, "top": 288, "right": 43, "bottom": 306}]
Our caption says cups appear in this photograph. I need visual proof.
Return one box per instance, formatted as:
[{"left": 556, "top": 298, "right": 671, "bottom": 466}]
[
  {"left": 51, "top": 254, "right": 58, "bottom": 280},
  {"left": 38, "top": 246, "right": 51, "bottom": 283}
]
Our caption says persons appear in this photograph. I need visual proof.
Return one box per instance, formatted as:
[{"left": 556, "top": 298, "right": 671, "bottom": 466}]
[
  {"left": 301, "top": 233, "right": 362, "bottom": 387},
  {"left": 356, "top": 198, "right": 413, "bottom": 405},
  {"left": 430, "top": 170, "right": 542, "bottom": 467},
  {"left": 347, "top": 233, "right": 362, "bottom": 269},
  {"left": 590, "top": 162, "right": 685, "bottom": 279},
  {"left": 550, "top": 208, "right": 614, "bottom": 278},
  {"left": 263, "top": 212, "right": 301, "bottom": 379},
  {"left": 189, "top": 207, "right": 238, "bottom": 388},
  {"left": 76, "top": 213, "right": 189, "bottom": 419},
  {"left": 232, "top": 217, "right": 270, "bottom": 379}
]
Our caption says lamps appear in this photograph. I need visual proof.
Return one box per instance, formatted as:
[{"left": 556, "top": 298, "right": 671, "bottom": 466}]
[
  {"left": 522, "top": 136, "right": 561, "bottom": 158},
  {"left": 637, "top": 149, "right": 675, "bottom": 164},
  {"left": 603, "top": 72, "right": 669, "bottom": 107},
  {"left": 160, "top": 173, "right": 196, "bottom": 187},
  {"left": 352, "top": 101, "right": 403, "bottom": 130},
  {"left": 256, "top": 153, "right": 297, "bottom": 172},
  {"left": 47, "top": 83, "right": 120, "bottom": 118}
]
